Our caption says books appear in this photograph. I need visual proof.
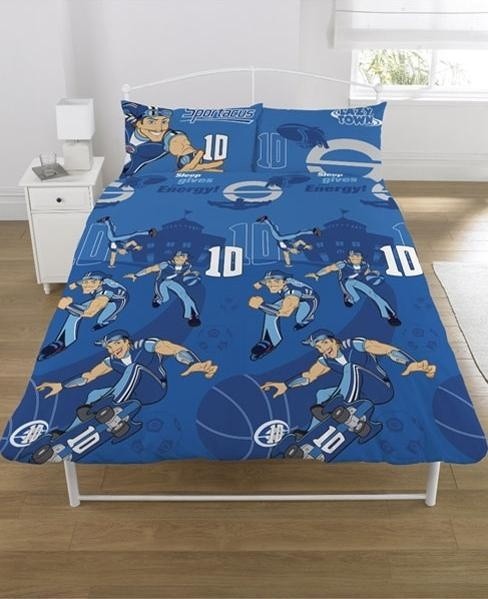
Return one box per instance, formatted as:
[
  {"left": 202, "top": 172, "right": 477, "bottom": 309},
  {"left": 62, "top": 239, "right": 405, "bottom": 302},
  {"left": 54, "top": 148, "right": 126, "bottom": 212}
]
[{"left": 32, "top": 162, "right": 70, "bottom": 183}]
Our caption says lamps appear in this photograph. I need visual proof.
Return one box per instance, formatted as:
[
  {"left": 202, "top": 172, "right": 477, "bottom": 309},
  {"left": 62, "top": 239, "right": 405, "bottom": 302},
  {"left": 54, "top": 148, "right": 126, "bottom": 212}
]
[{"left": 57, "top": 98, "right": 95, "bottom": 171}]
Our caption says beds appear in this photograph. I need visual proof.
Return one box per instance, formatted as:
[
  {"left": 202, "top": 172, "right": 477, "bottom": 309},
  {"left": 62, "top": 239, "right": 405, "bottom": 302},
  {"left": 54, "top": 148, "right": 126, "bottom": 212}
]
[{"left": 0, "top": 65, "right": 488, "bottom": 506}]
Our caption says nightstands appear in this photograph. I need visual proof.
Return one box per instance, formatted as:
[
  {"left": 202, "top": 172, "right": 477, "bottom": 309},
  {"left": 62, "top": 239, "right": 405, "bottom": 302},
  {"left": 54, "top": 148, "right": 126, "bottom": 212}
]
[{"left": 19, "top": 156, "right": 106, "bottom": 295}]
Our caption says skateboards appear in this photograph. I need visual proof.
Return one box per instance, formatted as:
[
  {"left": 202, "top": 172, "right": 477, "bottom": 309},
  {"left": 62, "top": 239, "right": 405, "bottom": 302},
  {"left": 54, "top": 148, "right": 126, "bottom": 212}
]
[
  {"left": 270, "top": 400, "right": 384, "bottom": 460},
  {"left": 31, "top": 392, "right": 144, "bottom": 465}
]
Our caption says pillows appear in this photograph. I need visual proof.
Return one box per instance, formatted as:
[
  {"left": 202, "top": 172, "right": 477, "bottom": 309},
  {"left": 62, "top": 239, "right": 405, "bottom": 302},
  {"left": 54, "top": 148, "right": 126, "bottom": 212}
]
[{"left": 118, "top": 96, "right": 387, "bottom": 187}]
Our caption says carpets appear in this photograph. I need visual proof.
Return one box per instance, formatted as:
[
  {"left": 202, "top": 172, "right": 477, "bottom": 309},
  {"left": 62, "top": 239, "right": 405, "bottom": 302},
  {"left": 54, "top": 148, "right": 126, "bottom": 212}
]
[{"left": 431, "top": 257, "right": 488, "bottom": 382}]
